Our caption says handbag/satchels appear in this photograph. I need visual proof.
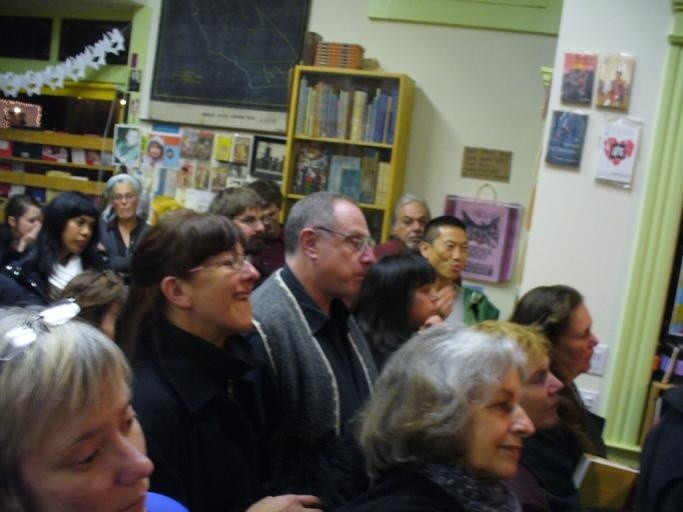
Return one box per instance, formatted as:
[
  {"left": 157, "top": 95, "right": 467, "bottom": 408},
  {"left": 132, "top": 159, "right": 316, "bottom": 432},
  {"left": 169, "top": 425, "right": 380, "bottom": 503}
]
[{"left": 444, "top": 185, "right": 521, "bottom": 284}]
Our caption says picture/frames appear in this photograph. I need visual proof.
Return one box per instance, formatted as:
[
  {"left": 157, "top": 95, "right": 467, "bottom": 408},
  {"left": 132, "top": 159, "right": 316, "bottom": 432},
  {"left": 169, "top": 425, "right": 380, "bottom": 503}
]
[
  {"left": 111, "top": 122, "right": 139, "bottom": 169},
  {"left": 248, "top": 133, "right": 287, "bottom": 181}
]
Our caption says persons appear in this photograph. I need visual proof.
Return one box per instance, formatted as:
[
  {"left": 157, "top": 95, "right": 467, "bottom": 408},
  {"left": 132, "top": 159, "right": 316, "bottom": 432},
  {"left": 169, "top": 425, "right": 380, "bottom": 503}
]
[
  {"left": 625, "top": 386, "right": 681, "bottom": 510},
  {"left": 0, "top": 172, "right": 608, "bottom": 511},
  {"left": 608, "top": 70, "right": 625, "bottom": 106},
  {"left": 140, "top": 136, "right": 167, "bottom": 168},
  {"left": 114, "top": 129, "right": 139, "bottom": 166}
]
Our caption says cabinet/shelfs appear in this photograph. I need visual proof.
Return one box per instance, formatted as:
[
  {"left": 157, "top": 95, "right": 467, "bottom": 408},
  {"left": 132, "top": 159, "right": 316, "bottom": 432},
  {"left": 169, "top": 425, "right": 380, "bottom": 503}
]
[
  {"left": 278, "top": 67, "right": 413, "bottom": 248},
  {"left": 0, "top": 74, "right": 124, "bottom": 228}
]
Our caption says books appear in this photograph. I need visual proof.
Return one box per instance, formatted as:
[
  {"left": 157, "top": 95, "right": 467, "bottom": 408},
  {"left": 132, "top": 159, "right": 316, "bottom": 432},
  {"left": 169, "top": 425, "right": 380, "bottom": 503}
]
[
  {"left": 291, "top": 75, "right": 398, "bottom": 206},
  {"left": 314, "top": 42, "right": 365, "bottom": 69}
]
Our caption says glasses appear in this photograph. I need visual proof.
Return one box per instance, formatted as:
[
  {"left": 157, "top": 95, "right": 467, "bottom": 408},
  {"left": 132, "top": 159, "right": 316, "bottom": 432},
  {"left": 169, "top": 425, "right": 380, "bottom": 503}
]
[
  {"left": 309, "top": 224, "right": 377, "bottom": 255},
  {"left": 183, "top": 252, "right": 249, "bottom": 274}
]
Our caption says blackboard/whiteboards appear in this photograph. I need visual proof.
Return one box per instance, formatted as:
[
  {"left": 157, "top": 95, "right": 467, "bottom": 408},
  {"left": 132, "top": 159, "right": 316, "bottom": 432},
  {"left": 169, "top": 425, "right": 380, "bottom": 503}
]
[{"left": 150, "top": 0, "right": 311, "bottom": 113}]
